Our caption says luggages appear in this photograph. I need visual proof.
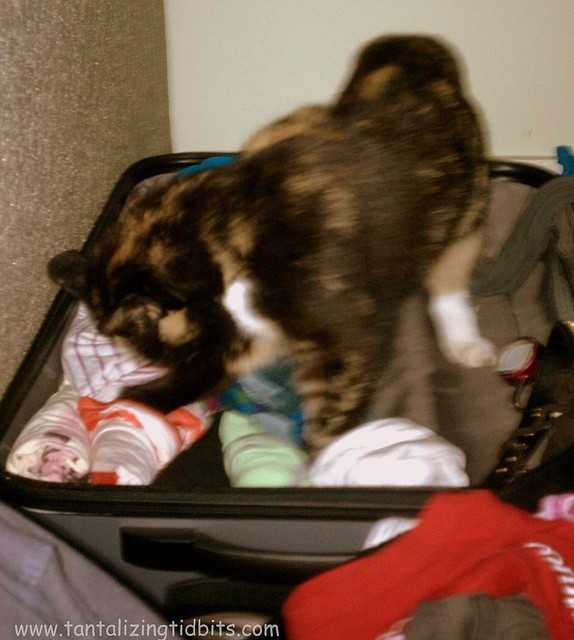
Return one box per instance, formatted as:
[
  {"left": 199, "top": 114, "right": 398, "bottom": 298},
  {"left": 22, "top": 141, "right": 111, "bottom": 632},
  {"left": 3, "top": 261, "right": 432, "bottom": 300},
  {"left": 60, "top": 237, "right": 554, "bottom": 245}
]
[{"left": 1, "top": 148, "right": 574, "bottom": 623}]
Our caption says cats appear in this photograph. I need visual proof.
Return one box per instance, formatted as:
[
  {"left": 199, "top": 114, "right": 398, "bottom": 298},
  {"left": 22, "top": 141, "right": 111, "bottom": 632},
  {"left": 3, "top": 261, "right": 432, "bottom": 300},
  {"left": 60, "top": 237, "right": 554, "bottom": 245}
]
[{"left": 46, "top": 34, "right": 499, "bottom": 462}]
